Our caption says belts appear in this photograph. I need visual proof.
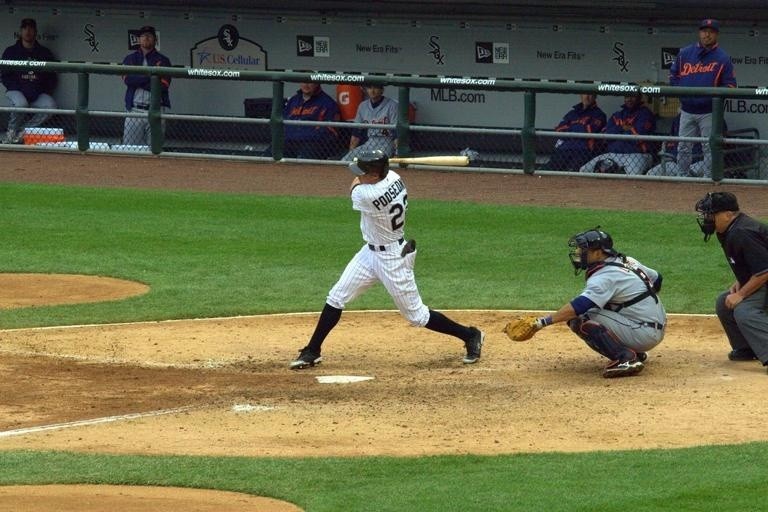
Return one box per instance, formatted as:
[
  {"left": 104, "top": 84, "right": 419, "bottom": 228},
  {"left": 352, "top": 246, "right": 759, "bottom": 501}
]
[
  {"left": 637, "top": 320, "right": 663, "bottom": 331},
  {"left": 130, "top": 102, "right": 151, "bottom": 111},
  {"left": 366, "top": 238, "right": 404, "bottom": 251}
]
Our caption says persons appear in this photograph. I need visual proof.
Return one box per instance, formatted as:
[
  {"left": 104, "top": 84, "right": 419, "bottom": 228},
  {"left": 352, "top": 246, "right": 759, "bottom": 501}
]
[
  {"left": 579, "top": 94, "right": 656, "bottom": 175},
  {"left": 289, "top": 151, "right": 484, "bottom": 375},
  {"left": 670, "top": 19, "right": 737, "bottom": 179},
  {"left": 264, "top": 81, "right": 341, "bottom": 160},
  {"left": 532, "top": 226, "right": 667, "bottom": 378},
  {"left": 0, "top": 18, "right": 58, "bottom": 145},
  {"left": 341, "top": 84, "right": 400, "bottom": 161},
  {"left": 695, "top": 191, "right": 768, "bottom": 374},
  {"left": 647, "top": 111, "right": 728, "bottom": 177},
  {"left": 122, "top": 26, "right": 171, "bottom": 146},
  {"left": 537, "top": 94, "right": 606, "bottom": 174}
]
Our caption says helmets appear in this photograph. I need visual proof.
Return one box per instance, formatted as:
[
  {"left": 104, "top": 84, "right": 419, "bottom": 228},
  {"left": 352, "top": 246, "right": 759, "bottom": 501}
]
[
  {"left": 567, "top": 229, "right": 618, "bottom": 270},
  {"left": 695, "top": 191, "right": 740, "bottom": 215},
  {"left": 348, "top": 149, "right": 390, "bottom": 178}
]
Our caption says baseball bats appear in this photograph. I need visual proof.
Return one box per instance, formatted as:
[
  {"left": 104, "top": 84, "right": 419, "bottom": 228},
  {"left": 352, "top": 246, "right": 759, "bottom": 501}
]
[{"left": 389, "top": 156, "right": 470, "bottom": 166}]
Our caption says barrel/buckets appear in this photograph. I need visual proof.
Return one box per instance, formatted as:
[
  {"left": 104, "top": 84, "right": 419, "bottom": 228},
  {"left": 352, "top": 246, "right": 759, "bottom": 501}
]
[
  {"left": 408, "top": 100, "right": 418, "bottom": 121},
  {"left": 337, "top": 83, "right": 363, "bottom": 119},
  {"left": 23, "top": 126, "right": 64, "bottom": 143}
]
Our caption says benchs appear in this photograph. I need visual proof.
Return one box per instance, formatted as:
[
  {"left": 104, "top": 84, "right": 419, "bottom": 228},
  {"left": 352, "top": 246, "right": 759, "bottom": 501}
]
[{"left": 0, "top": 103, "right": 760, "bottom": 174}]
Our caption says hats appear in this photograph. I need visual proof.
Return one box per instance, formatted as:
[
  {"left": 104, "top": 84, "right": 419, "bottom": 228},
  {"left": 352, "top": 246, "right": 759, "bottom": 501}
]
[
  {"left": 698, "top": 19, "right": 720, "bottom": 32},
  {"left": 19, "top": 17, "right": 38, "bottom": 29},
  {"left": 136, "top": 25, "right": 156, "bottom": 35},
  {"left": 593, "top": 158, "right": 619, "bottom": 173}
]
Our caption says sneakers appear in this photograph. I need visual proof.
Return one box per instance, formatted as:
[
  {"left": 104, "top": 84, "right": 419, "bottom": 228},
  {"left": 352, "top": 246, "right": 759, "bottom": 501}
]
[
  {"left": 602, "top": 351, "right": 648, "bottom": 377},
  {"left": 1, "top": 128, "right": 25, "bottom": 144},
  {"left": 287, "top": 347, "right": 323, "bottom": 370},
  {"left": 460, "top": 326, "right": 486, "bottom": 363},
  {"left": 727, "top": 347, "right": 758, "bottom": 361}
]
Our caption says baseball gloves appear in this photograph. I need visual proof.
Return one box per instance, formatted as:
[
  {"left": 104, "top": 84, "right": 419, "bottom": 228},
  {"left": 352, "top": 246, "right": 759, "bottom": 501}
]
[{"left": 502, "top": 315, "right": 539, "bottom": 341}]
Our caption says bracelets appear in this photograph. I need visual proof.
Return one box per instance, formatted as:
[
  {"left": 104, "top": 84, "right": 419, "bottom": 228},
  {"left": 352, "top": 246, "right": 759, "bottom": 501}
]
[{"left": 538, "top": 315, "right": 553, "bottom": 327}]
